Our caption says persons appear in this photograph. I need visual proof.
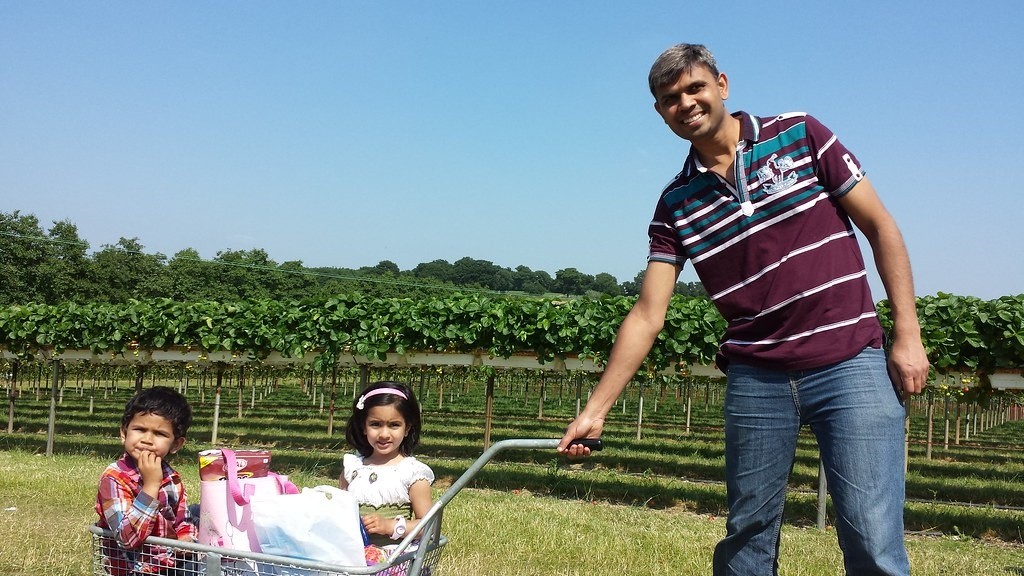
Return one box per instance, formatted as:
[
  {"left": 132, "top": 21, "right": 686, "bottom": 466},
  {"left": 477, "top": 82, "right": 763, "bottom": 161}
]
[
  {"left": 96, "top": 386, "right": 200, "bottom": 576},
  {"left": 557, "top": 43, "right": 929, "bottom": 576},
  {"left": 339, "top": 381, "right": 435, "bottom": 547}
]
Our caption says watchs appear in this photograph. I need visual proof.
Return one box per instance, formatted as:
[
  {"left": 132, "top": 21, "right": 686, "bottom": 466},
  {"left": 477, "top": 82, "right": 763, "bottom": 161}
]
[{"left": 389, "top": 516, "right": 406, "bottom": 540}]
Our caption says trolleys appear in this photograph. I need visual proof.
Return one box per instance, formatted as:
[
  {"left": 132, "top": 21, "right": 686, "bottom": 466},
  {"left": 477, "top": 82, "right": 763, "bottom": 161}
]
[{"left": 88, "top": 435, "right": 603, "bottom": 576}]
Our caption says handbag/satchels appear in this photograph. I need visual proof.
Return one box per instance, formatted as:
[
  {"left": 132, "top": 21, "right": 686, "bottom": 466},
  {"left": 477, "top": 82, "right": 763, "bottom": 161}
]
[
  {"left": 250, "top": 485, "right": 369, "bottom": 576},
  {"left": 199, "top": 449, "right": 299, "bottom": 561}
]
